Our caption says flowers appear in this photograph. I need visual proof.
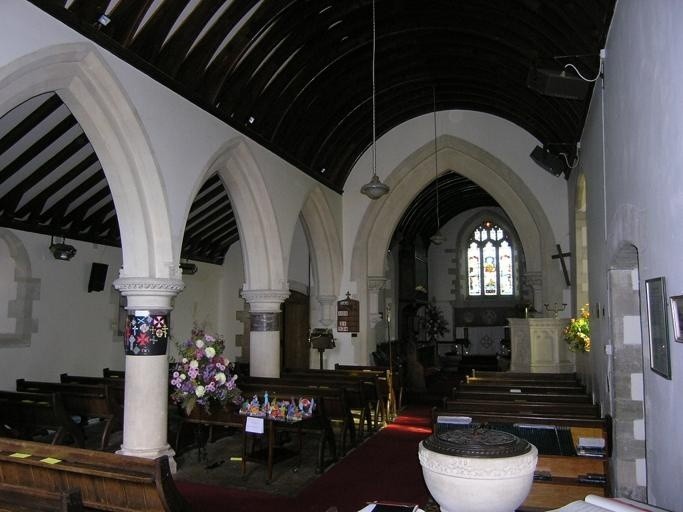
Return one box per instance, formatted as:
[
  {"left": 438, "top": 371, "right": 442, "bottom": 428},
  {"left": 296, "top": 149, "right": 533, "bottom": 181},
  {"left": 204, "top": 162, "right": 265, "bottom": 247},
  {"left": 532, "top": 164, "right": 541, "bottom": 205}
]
[
  {"left": 165, "top": 321, "right": 244, "bottom": 418},
  {"left": 557, "top": 302, "right": 592, "bottom": 354}
]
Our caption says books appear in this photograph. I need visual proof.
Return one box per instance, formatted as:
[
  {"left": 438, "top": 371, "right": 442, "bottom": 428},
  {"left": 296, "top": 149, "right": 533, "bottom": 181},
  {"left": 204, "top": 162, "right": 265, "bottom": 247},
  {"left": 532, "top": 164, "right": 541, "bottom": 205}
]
[{"left": 544, "top": 494, "right": 673, "bottom": 512}]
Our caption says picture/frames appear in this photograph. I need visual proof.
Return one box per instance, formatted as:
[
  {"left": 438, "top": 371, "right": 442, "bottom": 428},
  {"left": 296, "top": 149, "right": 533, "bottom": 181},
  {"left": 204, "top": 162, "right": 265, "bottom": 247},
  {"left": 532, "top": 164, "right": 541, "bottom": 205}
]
[
  {"left": 668, "top": 294, "right": 683, "bottom": 344},
  {"left": 643, "top": 274, "right": 672, "bottom": 382}
]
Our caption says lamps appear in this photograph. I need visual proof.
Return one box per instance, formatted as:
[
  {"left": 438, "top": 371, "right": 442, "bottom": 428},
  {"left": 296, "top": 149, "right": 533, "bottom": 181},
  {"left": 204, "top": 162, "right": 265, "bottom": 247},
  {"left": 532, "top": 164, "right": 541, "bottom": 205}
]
[
  {"left": 559, "top": 54, "right": 604, "bottom": 83},
  {"left": 424, "top": 83, "right": 451, "bottom": 246},
  {"left": 354, "top": 1, "right": 394, "bottom": 201}
]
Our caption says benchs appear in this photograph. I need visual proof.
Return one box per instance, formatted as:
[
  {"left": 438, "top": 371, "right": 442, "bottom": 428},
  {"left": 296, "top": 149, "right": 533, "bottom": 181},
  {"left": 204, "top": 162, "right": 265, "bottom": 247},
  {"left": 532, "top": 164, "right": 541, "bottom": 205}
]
[
  {"left": 1, "top": 364, "right": 398, "bottom": 510},
  {"left": 366, "top": 369, "right": 613, "bottom": 511}
]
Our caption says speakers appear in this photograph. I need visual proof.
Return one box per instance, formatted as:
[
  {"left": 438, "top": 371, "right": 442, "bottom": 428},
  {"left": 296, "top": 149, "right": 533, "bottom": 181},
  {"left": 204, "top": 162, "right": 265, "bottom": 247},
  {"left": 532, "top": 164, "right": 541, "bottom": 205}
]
[
  {"left": 88, "top": 262, "right": 109, "bottom": 292},
  {"left": 529, "top": 144, "right": 566, "bottom": 178}
]
